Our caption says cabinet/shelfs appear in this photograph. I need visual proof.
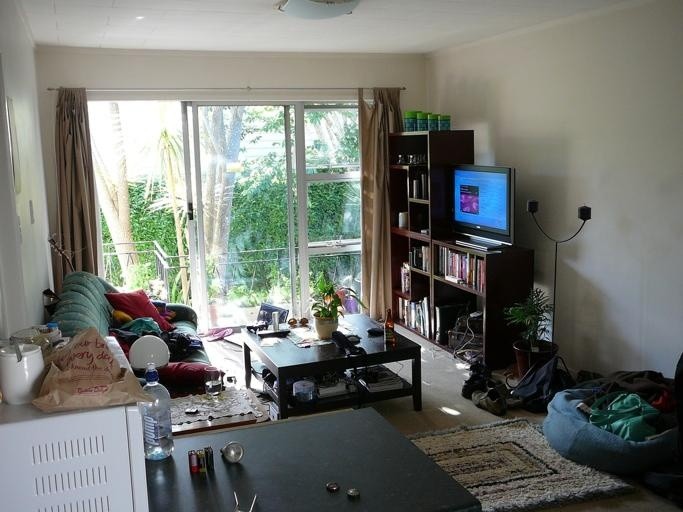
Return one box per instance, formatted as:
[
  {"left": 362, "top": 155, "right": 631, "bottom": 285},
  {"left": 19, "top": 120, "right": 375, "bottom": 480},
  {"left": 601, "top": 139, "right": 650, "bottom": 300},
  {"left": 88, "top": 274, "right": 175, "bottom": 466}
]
[
  {"left": 427, "top": 236, "right": 540, "bottom": 368},
  {"left": 384, "top": 127, "right": 475, "bottom": 346}
]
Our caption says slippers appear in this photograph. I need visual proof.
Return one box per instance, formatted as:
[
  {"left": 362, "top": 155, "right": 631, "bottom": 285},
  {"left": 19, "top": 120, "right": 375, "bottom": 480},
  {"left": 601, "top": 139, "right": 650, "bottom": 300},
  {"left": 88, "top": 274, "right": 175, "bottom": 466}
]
[{"left": 207, "top": 328, "right": 233, "bottom": 342}]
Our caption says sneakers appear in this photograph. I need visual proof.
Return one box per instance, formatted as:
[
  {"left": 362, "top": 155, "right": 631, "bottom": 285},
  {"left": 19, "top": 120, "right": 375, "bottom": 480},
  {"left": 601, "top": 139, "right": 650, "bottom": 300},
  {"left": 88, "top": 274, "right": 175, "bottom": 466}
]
[
  {"left": 471, "top": 391, "right": 506, "bottom": 416},
  {"left": 486, "top": 380, "right": 521, "bottom": 407}
]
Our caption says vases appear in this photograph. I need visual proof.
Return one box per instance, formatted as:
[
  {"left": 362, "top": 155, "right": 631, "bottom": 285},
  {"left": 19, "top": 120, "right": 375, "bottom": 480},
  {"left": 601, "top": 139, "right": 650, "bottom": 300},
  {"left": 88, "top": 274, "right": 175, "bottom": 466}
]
[{"left": 312, "top": 314, "right": 338, "bottom": 338}]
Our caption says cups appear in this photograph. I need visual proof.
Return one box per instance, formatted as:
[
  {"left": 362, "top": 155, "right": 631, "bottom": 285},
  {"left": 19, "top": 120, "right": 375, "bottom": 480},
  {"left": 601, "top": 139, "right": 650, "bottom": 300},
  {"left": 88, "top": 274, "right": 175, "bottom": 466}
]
[
  {"left": 259, "top": 311, "right": 267, "bottom": 321},
  {"left": 397, "top": 212, "right": 407, "bottom": 227},
  {"left": 9, "top": 327, "right": 61, "bottom": 356},
  {"left": 0, "top": 344, "right": 45, "bottom": 406},
  {"left": 203, "top": 366, "right": 222, "bottom": 396}
]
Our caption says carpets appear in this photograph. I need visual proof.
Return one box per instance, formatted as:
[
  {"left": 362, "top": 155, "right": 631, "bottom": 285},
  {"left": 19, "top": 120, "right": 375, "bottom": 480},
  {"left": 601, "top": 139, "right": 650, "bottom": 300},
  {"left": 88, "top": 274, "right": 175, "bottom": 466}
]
[{"left": 389, "top": 414, "right": 640, "bottom": 511}]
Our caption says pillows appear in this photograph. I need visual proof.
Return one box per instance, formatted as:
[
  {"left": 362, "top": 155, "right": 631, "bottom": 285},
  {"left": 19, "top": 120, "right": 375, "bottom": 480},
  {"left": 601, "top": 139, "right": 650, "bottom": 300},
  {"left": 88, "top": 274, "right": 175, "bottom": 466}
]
[
  {"left": 100, "top": 289, "right": 175, "bottom": 331},
  {"left": 145, "top": 358, "right": 219, "bottom": 396}
]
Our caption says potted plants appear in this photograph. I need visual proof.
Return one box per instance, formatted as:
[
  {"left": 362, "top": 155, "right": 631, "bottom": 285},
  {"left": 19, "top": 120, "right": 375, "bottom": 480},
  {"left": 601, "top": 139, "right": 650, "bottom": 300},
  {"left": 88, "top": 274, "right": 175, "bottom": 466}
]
[{"left": 499, "top": 287, "right": 563, "bottom": 383}]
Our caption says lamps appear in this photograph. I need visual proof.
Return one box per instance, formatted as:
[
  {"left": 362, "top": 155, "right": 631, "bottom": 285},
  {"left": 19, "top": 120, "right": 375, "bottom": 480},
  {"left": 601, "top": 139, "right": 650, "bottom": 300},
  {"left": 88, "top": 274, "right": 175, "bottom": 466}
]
[
  {"left": 272, "top": 0, "right": 363, "bottom": 20},
  {"left": 521, "top": 196, "right": 595, "bottom": 396}
]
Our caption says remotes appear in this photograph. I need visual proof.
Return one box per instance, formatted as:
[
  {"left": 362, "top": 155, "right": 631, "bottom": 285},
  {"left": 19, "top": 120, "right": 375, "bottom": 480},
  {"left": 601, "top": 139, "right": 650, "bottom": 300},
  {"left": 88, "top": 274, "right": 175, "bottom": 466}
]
[
  {"left": 258, "top": 329, "right": 290, "bottom": 337},
  {"left": 469, "top": 311, "right": 482, "bottom": 317}
]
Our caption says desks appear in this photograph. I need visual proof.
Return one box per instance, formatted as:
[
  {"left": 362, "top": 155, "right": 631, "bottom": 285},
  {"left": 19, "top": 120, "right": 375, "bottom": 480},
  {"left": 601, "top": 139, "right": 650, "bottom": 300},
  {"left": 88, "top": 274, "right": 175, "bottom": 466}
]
[
  {"left": 162, "top": 389, "right": 271, "bottom": 433},
  {"left": 142, "top": 406, "right": 488, "bottom": 512}
]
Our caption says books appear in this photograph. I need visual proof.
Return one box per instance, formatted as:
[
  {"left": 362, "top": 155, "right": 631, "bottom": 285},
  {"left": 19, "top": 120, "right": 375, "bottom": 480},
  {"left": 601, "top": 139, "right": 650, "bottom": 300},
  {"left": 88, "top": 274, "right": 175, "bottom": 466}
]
[
  {"left": 317, "top": 365, "right": 404, "bottom": 398},
  {"left": 398, "top": 245, "right": 484, "bottom": 346}
]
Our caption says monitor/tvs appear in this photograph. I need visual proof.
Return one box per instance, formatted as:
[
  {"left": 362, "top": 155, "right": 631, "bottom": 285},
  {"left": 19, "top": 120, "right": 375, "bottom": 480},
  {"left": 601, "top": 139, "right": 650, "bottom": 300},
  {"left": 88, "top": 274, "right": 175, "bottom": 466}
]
[{"left": 447, "top": 164, "right": 515, "bottom": 252}]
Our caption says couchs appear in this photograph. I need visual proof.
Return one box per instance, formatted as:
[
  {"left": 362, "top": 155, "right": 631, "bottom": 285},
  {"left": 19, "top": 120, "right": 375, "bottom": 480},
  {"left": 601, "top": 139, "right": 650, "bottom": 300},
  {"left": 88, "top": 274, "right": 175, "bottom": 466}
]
[{"left": 44, "top": 267, "right": 222, "bottom": 397}]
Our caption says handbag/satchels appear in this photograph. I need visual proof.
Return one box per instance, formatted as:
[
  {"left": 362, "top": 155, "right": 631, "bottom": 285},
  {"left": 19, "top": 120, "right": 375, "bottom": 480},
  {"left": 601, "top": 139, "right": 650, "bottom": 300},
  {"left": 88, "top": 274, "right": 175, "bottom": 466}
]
[
  {"left": 514, "top": 358, "right": 575, "bottom": 413},
  {"left": 588, "top": 391, "right": 661, "bottom": 442}
]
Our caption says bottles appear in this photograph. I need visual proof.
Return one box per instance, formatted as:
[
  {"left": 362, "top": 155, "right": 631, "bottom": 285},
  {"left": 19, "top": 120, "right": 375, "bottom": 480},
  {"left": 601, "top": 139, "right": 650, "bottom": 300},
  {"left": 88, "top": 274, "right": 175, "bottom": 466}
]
[
  {"left": 397, "top": 153, "right": 424, "bottom": 164},
  {"left": 421, "top": 173, "right": 427, "bottom": 200},
  {"left": 383, "top": 309, "right": 394, "bottom": 343},
  {"left": 271, "top": 312, "right": 279, "bottom": 331},
  {"left": 138, "top": 361, "right": 175, "bottom": 460},
  {"left": 412, "top": 178, "right": 420, "bottom": 199}
]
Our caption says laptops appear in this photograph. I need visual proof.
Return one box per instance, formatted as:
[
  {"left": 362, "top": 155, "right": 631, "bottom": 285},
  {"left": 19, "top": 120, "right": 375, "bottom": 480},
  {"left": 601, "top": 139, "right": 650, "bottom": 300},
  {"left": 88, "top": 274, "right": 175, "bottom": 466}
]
[{"left": 223, "top": 302, "right": 289, "bottom": 352}]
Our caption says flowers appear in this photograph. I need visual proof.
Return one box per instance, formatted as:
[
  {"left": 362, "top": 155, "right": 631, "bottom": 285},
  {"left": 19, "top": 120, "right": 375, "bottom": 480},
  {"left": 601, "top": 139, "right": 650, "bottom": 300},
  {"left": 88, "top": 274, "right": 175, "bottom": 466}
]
[{"left": 305, "top": 262, "right": 365, "bottom": 317}]
